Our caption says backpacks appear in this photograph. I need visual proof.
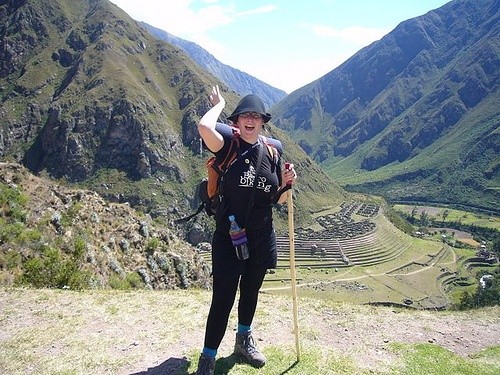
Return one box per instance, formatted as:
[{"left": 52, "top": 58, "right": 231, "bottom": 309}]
[{"left": 205, "top": 127, "right": 280, "bottom": 207}]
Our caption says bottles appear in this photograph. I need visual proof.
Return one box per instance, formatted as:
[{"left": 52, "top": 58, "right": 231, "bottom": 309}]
[{"left": 228, "top": 215, "right": 249, "bottom": 260}]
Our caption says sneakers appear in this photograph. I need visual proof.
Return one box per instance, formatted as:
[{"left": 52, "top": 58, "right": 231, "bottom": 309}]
[
  {"left": 195, "top": 354, "right": 217, "bottom": 375},
  {"left": 232, "top": 330, "right": 266, "bottom": 369}
]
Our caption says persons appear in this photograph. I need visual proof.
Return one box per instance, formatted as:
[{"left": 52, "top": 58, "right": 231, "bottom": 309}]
[{"left": 192, "top": 83, "right": 298, "bottom": 375}]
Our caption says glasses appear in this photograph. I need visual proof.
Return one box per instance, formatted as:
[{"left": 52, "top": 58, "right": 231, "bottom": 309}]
[{"left": 238, "top": 111, "right": 263, "bottom": 120}]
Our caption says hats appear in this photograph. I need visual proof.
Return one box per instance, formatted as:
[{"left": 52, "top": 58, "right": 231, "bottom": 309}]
[{"left": 225, "top": 93, "right": 271, "bottom": 124}]
